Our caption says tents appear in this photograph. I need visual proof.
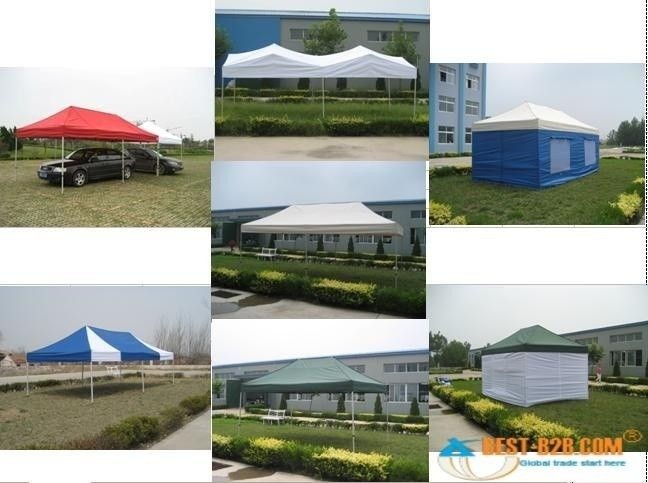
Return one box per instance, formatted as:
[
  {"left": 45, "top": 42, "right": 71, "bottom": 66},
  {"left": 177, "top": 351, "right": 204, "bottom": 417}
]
[
  {"left": 472, "top": 101, "right": 600, "bottom": 189},
  {"left": 481, "top": 324, "right": 589, "bottom": 408}
]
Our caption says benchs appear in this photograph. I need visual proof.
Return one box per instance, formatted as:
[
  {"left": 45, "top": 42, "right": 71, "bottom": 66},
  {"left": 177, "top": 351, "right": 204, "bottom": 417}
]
[
  {"left": 262, "top": 408, "right": 286, "bottom": 426},
  {"left": 434, "top": 376, "right": 450, "bottom": 386},
  {"left": 258, "top": 247, "right": 279, "bottom": 261}
]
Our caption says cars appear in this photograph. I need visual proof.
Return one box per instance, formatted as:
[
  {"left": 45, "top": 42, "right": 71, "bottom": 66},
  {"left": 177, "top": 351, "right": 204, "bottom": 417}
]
[
  {"left": 36, "top": 147, "right": 137, "bottom": 188},
  {"left": 118, "top": 147, "right": 185, "bottom": 177}
]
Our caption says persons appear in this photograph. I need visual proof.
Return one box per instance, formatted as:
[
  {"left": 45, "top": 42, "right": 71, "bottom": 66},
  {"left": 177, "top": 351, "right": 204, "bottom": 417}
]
[{"left": 596, "top": 364, "right": 601, "bottom": 383}]
